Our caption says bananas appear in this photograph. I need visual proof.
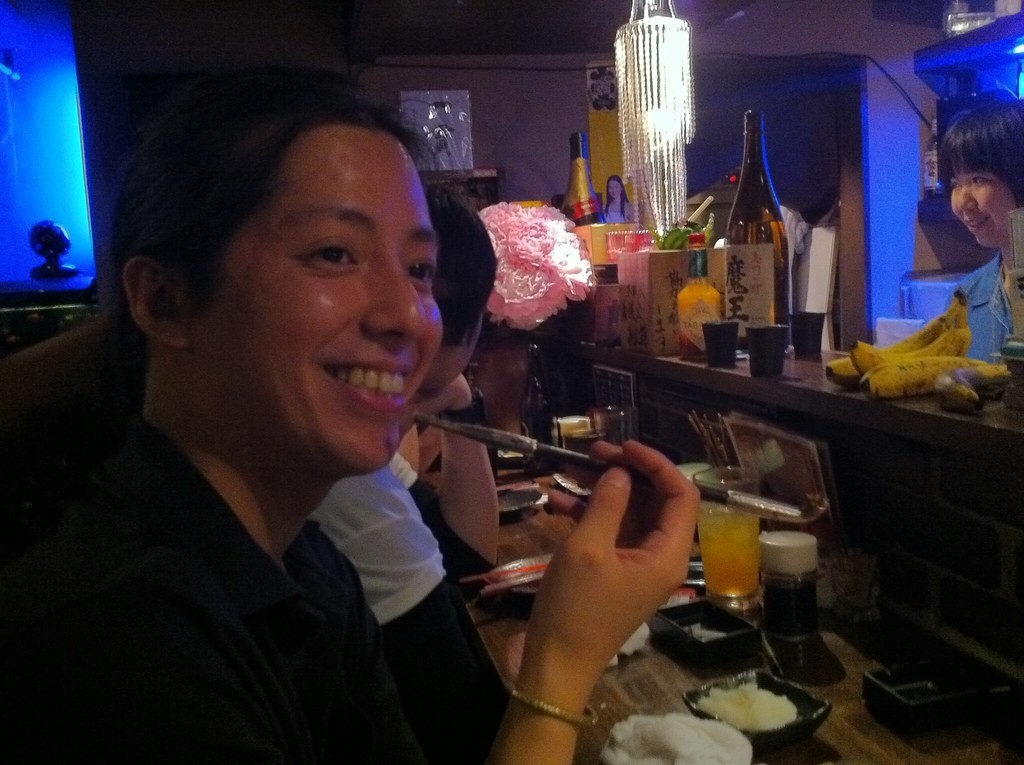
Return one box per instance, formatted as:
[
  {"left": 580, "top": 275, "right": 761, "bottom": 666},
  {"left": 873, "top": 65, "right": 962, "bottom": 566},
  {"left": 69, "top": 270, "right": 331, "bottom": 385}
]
[{"left": 826, "top": 289, "right": 1012, "bottom": 401}]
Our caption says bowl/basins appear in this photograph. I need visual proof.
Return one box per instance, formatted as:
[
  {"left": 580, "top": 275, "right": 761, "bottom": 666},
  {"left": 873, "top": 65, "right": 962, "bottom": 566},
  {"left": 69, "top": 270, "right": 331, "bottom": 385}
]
[
  {"left": 497, "top": 488, "right": 542, "bottom": 523},
  {"left": 601, "top": 721, "right": 754, "bottom": 765},
  {"left": 683, "top": 669, "right": 831, "bottom": 756}
]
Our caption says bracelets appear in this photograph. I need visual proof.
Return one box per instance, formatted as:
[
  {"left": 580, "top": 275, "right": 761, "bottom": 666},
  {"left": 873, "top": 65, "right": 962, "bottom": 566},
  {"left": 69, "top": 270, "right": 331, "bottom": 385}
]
[{"left": 509, "top": 684, "right": 598, "bottom": 729}]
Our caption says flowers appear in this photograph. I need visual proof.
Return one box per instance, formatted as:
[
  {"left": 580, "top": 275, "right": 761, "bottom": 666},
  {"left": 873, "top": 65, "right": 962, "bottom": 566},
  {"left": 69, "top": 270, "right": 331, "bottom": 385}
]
[{"left": 481, "top": 199, "right": 593, "bottom": 332}]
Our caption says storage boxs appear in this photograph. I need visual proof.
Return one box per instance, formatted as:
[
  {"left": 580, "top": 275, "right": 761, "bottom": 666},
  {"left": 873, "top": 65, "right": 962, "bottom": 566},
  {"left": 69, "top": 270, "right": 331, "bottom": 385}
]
[
  {"left": 573, "top": 222, "right": 638, "bottom": 264},
  {"left": 648, "top": 596, "right": 762, "bottom": 677},
  {"left": 865, "top": 654, "right": 1012, "bottom": 736},
  {"left": 614, "top": 244, "right": 778, "bottom": 355}
]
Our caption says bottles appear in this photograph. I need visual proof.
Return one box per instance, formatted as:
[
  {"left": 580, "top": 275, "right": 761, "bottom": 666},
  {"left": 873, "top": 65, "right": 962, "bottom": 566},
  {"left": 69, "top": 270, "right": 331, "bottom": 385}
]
[
  {"left": 558, "top": 132, "right": 605, "bottom": 226},
  {"left": 922, "top": 119, "right": 943, "bottom": 201},
  {"left": 521, "top": 346, "right": 557, "bottom": 477},
  {"left": 678, "top": 233, "right": 722, "bottom": 362},
  {"left": 723, "top": 109, "right": 793, "bottom": 365}
]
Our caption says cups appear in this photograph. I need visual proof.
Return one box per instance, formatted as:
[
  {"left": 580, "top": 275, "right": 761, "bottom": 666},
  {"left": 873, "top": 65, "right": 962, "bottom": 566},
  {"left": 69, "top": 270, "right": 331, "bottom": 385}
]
[
  {"left": 757, "top": 530, "right": 819, "bottom": 644},
  {"left": 745, "top": 325, "right": 790, "bottom": 380},
  {"left": 692, "top": 464, "right": 760, "bottom": 611},
  {"left": 558, "top": 407, "right": 636, "bottom": 491},
  {"left": 701, "top": 322, "right": 740, "bottom": 369},
  {"left": 788, "top": 311, "right": 825, "bottom": 353}
]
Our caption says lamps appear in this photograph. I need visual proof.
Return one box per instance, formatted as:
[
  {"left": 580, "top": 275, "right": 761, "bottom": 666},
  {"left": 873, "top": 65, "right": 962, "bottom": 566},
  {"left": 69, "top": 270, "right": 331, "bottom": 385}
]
[{"left": 613, "top": 0, "right": 696, "bottom": 237}]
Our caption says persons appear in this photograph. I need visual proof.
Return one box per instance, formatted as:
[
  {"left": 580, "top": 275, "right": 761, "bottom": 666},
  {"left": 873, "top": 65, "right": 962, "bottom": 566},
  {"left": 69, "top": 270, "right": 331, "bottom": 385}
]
[
  {"left": 0, "top": 63, "right": 702, "bottom": 764},
  {"left": 936, "top": 100, "right": 1024, "bottom": 363},
  {"left": 602, "top": 174, "right": 632, "bottom": 223}
]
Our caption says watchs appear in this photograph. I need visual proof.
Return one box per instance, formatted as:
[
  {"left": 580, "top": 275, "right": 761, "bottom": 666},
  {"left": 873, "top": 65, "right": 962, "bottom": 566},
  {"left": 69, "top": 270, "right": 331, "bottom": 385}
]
[{"left": 438, "top": 387, "right": 486, "bottom": 424}]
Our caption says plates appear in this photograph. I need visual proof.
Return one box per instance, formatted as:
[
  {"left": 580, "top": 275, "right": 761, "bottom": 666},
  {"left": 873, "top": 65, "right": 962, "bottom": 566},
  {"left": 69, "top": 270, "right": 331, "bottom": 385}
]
[{"left": 490, "top": 554, "right": 556, "bottom": 596}]
